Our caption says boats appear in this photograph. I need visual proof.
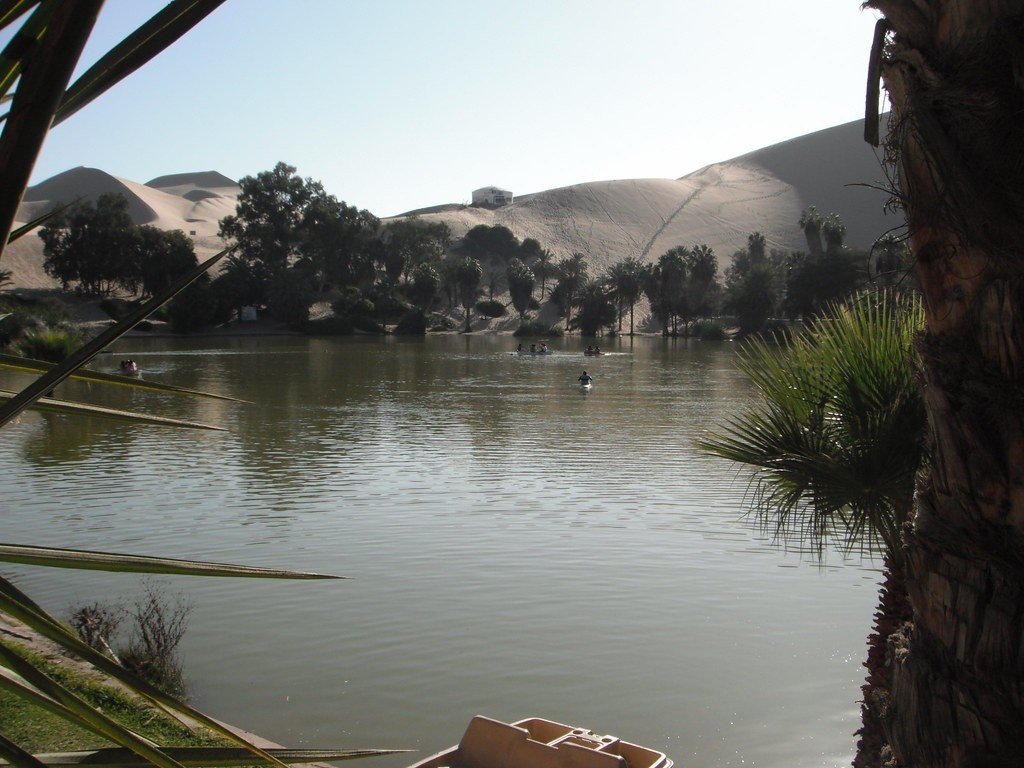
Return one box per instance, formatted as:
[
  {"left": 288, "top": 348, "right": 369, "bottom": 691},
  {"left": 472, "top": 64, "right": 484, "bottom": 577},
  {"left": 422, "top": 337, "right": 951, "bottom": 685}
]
[
  {"left": 112, "top": 368, "right": 142, "bottom": 378},
  {"left": 577, "top": 381, "right": 591, "bottom": 392},
  {"left": 506, "top": 349, "right": 555, "bottom": 356},
  {"left": 409, "top": 713, "right": 674, "bottom": 768},
  {"left": 584, "top": 349, "right": 605, "bottom": 356}
]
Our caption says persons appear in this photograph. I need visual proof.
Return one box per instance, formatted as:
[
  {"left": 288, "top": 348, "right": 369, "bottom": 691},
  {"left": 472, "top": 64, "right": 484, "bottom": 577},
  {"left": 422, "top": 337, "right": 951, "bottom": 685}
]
[
  {"left": 577, "top": 370, "right": 593, "bottom": 385},
  {"left": 120, "top": 359, "right": 138, "bottom": 372},
  {"left": 588, "top": 346, "right": 593, "bottom": 351},
  {"left": 517, "top": 343, "right": 547, "bottom": 353},
  {"left": 595, "top": 345, "right": 601, "bottom": 351}
]
